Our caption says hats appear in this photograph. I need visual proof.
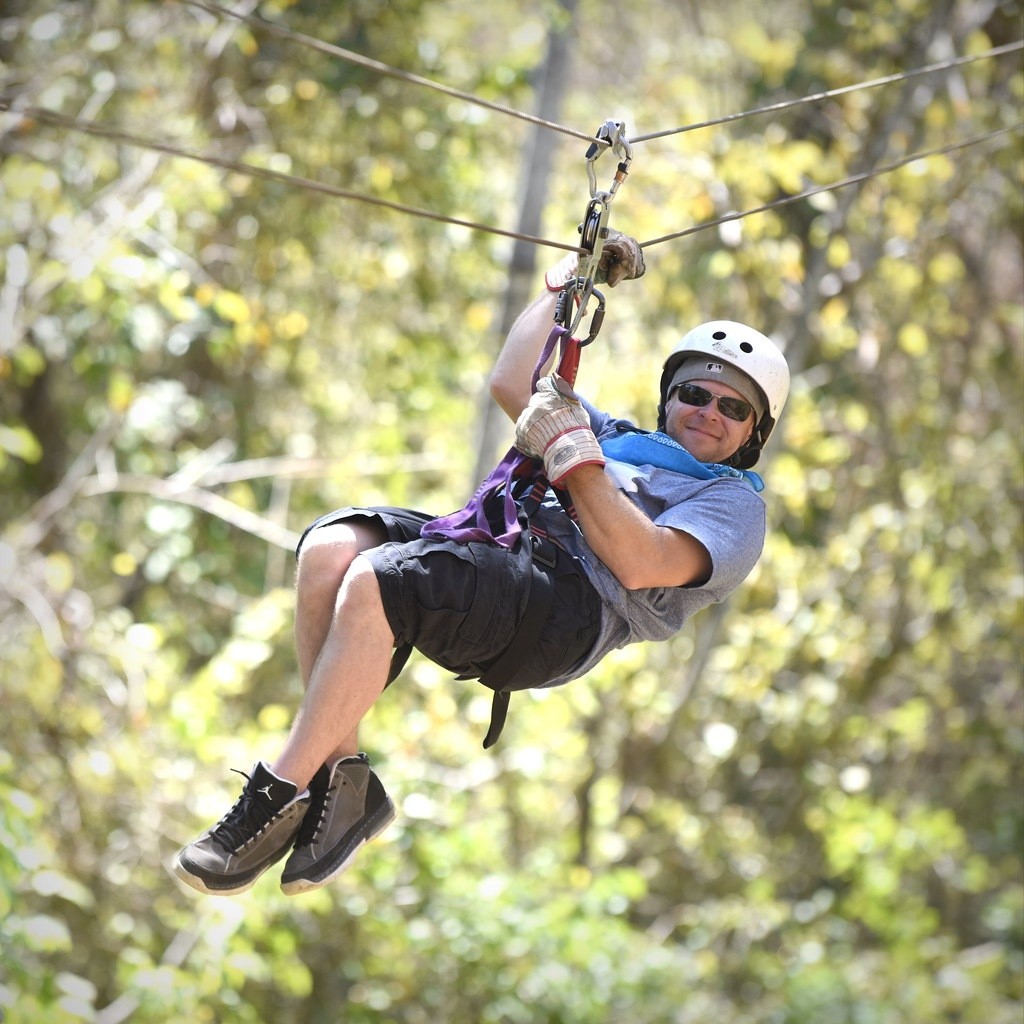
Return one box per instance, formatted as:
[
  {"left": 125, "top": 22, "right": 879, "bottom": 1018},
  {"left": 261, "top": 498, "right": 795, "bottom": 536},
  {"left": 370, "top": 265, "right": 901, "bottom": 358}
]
[{"left": 666, "top": 359, "right": 767, "bottom": 427}]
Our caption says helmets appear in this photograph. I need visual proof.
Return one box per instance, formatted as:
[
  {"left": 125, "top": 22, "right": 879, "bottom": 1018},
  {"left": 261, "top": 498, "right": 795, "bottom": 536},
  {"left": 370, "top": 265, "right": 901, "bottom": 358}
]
[{"left": 657, "top": 320, "right": 789, "bottom": 469}]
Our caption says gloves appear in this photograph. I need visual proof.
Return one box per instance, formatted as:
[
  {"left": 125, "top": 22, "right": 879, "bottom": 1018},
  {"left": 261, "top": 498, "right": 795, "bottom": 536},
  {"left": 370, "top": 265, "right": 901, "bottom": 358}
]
[
  {"left": 545, "top": 226, "right": 645, "bottom": 291},
  {"left": 516, "top": 377, "right": 606, "bottom": 489}
]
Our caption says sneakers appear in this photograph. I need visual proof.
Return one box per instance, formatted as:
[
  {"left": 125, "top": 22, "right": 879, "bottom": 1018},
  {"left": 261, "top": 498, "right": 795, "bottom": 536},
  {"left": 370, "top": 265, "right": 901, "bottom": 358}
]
[
  {"left": 280, "top": 752, "right": 397, "bottom": 895},
  {"left": 172, "top": 755, "right": 311, "bottom": 895}
]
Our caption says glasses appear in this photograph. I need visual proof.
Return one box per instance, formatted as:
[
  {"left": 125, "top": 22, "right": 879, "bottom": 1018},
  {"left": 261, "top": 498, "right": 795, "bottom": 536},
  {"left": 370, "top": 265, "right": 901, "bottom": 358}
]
[{"left": 676, "top": 381, "right": 756, "bottom": 422}]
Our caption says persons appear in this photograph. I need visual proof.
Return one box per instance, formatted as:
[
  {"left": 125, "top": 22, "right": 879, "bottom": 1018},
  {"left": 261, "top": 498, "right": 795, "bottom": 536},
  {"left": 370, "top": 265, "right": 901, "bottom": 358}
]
[{"left": 168, "top": 230, "right": 794, "bottom": 899}]
